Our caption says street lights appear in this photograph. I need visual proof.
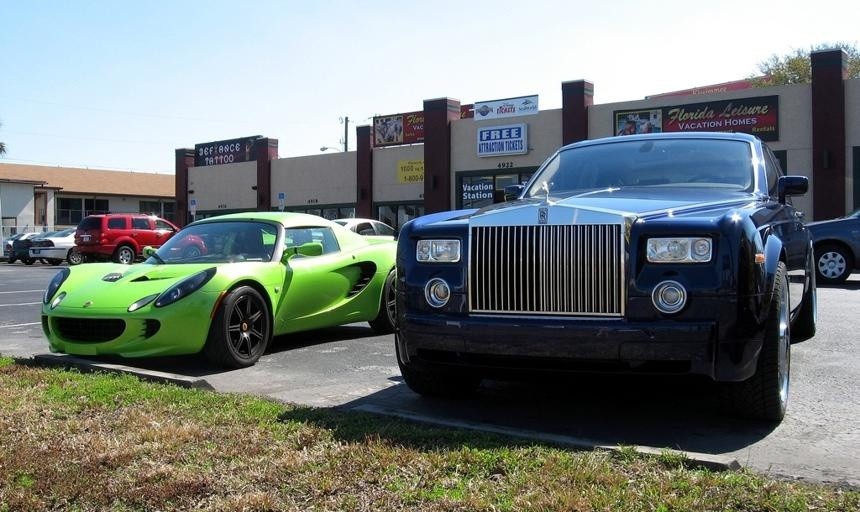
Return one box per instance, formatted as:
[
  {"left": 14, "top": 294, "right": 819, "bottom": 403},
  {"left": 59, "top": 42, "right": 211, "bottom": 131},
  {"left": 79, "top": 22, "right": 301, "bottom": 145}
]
[{"left": 320, "top": 146, "right": 341, "bottom": 153}]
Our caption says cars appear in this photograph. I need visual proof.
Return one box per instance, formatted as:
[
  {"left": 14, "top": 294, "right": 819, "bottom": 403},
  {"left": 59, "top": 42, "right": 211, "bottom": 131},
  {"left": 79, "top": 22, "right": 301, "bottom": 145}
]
[
  {"left": 802, "top": 201, "right": 860, "bottom": 289},
  {"left": 1, "top": 227, "right": 87, "bottom": 266},
  {"left": 298, "top": 217, "right": 396, "bottom": 243}
]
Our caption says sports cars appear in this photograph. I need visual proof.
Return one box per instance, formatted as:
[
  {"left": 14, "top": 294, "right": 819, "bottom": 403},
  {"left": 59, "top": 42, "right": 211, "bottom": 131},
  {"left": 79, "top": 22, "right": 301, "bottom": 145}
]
[{"left": 40, "top": 211, "right": 400, "bottom": 369}]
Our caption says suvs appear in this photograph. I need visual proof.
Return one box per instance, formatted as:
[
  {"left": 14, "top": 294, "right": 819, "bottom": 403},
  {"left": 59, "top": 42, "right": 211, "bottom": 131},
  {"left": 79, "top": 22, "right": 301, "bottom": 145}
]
[
  {"left": 390, "top": 131, "right": 815, "bottom": 426},
  {"left": 74, "top": 212, "right": 207, "bottom": 265}
]
